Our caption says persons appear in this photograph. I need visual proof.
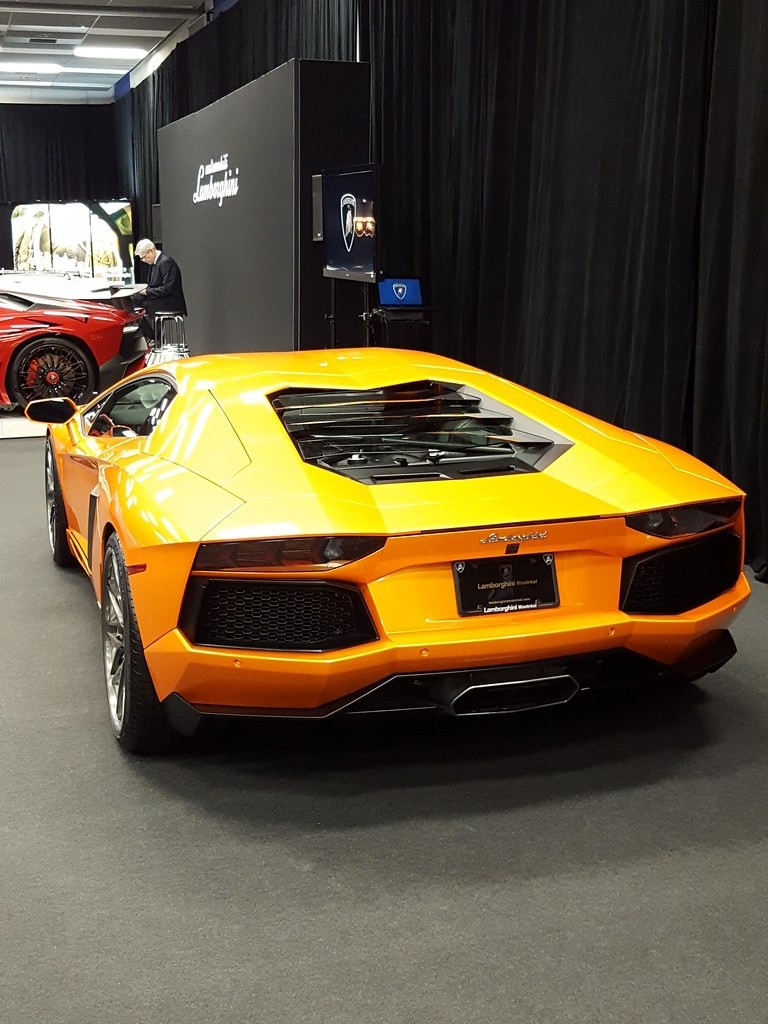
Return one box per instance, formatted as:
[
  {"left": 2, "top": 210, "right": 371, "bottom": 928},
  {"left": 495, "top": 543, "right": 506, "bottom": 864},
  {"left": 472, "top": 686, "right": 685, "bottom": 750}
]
[{"left": 133, "top": 238, "right": 188, "bottom": 346}]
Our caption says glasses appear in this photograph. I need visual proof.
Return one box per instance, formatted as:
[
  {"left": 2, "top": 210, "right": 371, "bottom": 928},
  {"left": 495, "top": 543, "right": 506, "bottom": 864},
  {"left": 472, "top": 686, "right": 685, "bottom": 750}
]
[{"left": 140, "top": 252, "right": 149, "bottom": 262}]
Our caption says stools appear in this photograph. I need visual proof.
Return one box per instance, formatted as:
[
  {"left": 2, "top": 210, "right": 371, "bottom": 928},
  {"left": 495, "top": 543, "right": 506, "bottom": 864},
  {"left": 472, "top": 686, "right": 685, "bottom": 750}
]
[{"left": 152, "top": 311, "right": 190, "bottom": 353}]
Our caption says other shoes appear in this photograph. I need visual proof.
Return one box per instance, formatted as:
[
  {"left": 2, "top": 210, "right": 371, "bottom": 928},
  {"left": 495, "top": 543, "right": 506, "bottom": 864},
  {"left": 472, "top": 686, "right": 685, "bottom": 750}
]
[{"left": 147, "top": 339, "right": 160, "bottom": 347}]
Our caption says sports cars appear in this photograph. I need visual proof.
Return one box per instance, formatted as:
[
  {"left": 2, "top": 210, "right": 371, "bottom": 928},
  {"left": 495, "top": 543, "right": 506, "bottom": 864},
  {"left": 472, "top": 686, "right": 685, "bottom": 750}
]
[
  {"left": 24, "top": 347, "right": 750, "bottom": 754},
  {"left": 1, "top": 281, "right": 151, "bottom": 416}
]
